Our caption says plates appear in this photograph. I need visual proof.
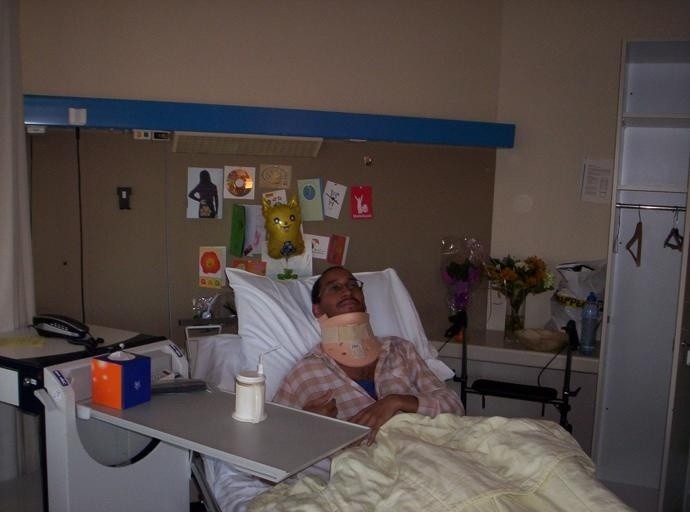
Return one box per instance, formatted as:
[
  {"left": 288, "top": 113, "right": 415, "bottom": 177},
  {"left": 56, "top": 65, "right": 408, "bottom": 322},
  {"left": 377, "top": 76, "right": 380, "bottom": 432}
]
[{"left": 512, "top": 327, "right": 569, "bottom": 350}]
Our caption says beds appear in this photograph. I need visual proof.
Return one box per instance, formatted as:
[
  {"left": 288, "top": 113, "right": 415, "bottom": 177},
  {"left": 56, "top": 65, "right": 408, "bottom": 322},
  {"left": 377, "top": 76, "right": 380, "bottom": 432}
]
[{"left": 181, "top": 316, "right": 637, "bottom": 511}]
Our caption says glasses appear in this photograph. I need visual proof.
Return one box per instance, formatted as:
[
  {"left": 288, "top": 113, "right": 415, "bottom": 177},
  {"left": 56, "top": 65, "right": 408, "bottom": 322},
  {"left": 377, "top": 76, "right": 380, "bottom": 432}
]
[{"left": 317, "top": 279, "right": 364, "bottom": 302}]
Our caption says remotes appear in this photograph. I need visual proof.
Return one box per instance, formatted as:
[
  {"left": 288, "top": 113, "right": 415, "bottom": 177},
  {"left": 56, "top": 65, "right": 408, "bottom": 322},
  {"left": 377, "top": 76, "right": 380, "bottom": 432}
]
[{"left": 152, "top": 379, "right": 207, "bottom": 394}]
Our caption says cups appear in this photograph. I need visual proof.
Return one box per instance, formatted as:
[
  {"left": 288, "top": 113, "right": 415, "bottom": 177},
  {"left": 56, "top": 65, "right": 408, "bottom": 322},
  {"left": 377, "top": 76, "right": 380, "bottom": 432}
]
[{"left": 232, "top": 371, "right": 269, "bottom": 423}]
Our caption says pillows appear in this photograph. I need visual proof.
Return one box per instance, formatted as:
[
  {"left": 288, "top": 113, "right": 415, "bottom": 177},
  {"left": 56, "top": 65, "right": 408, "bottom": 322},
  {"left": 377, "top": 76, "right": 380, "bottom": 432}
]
[{"left": 229, "top": 262, "right": 437, "bottom": 404}]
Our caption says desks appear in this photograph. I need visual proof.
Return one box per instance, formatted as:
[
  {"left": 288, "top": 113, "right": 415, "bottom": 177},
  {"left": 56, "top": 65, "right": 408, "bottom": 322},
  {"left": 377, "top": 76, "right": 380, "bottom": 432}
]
[
  {"left": 1, "top": 322, "right": 374, "bottom": 512},
  {"left": 427, "top": 326, "right": 600, "bottom": 459}
]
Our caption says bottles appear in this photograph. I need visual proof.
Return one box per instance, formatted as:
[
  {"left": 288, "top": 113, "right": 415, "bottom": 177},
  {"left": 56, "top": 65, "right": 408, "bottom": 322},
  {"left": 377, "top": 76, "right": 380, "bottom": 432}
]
[{"left": 579, "top": 291, "right": 603, "bottom": 353}]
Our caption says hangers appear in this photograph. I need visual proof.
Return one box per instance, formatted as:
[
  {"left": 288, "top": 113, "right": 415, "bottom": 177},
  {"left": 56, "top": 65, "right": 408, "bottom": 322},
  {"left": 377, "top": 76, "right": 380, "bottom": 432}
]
[
  {"left": 663, "top": 202, "right": 684, "bottom": 253},
  {"left": 625, "top": 202, "right": 644, "bottom": 267}
]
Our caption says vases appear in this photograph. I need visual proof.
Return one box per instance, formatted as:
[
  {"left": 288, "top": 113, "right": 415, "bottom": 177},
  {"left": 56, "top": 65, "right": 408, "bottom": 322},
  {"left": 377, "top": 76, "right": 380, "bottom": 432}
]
[{"left": 504, "top": 293, "right": 527, "bottom": 330}]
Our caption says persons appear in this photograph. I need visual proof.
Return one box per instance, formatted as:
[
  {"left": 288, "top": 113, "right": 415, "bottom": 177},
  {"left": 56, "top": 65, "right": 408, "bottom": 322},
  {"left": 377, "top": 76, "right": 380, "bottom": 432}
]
[
  {"left": 258, "top": 265, "right": 465, "bottom": 486},
  {"left": 188, "top": 170, "right": 218, "bottom": 218}
]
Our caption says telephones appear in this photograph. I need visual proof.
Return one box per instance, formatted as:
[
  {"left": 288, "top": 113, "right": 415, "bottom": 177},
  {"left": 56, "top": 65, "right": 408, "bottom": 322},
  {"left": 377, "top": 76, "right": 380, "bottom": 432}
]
[{"left": 31, "top": 314, "right": 89, "bottom": 338}]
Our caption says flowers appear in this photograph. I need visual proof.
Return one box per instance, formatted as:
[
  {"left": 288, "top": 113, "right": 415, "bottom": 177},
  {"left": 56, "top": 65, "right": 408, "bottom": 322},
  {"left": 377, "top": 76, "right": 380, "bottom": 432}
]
[
  {"left": 443, "top": 259, "right": 481, "bottom": 312},
  {"left": 480, "top": 253, "right": 556, "bottom": 326}
]
